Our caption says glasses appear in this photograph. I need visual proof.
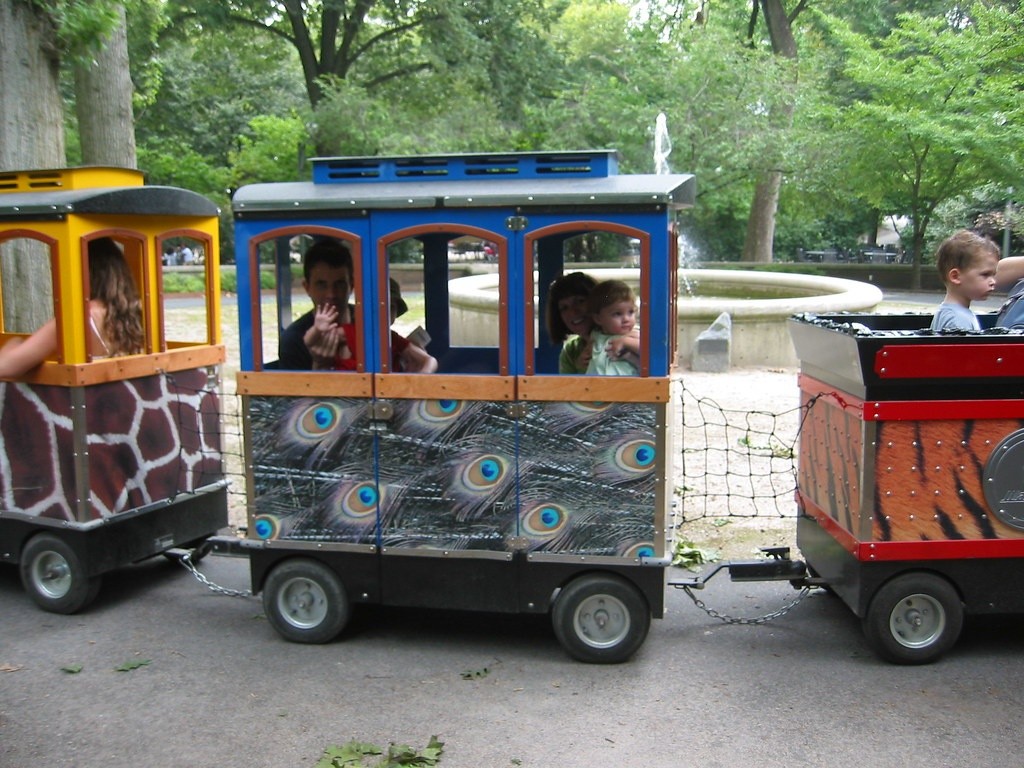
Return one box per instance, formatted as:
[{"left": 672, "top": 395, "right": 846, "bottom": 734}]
[{"left": 548, "top": 272, "right": 586, "bottom": 290}]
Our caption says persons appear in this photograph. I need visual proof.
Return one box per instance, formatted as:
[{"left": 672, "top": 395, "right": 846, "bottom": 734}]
[
  {"left": 166, "top": 243, "right": 194, "bottom": 266},
  {"left": 280, "top": 242, "right": 426, "bottom": 373},
  {"left": 545, "top": 272, "right": 640, "bottom": 375},
  {"left": 995, "top": 256, "right": 1024, "bottom": 329},
  {"left": 930, "top": 231, "right": 1000, "bottom": 330},
  {"left": 304, "top": 279, "right": 438, "bottom": 373},
  {"left": 484, "top": 242, "right": 495, "bottom": 262},
  {"left": 0, "top": 235, "right": 144, "bottom": 380}
]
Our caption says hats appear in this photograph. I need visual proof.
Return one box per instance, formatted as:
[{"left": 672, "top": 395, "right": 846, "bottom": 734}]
[{"left": 390, "top": 277, "right": 408, "bottom": 318}]
